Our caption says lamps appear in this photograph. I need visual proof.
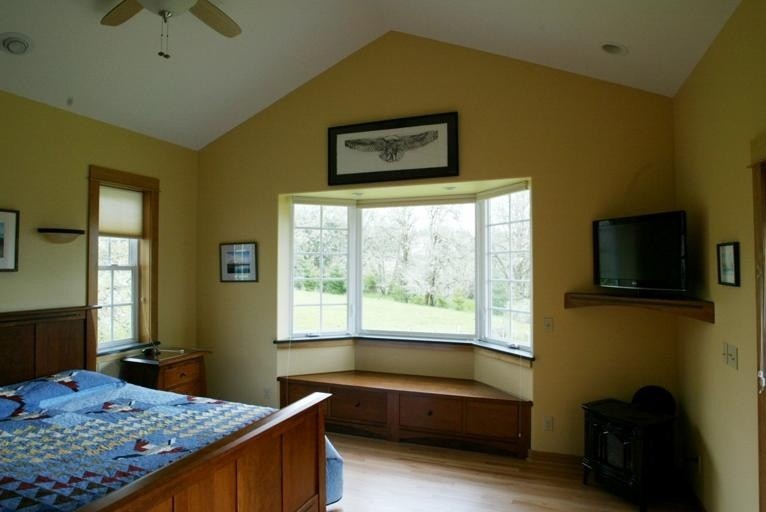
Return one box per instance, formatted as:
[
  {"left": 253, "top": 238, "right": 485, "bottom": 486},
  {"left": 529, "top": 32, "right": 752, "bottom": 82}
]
[{"left": 38, "top": 228, "right": 86, "bottom": 244}]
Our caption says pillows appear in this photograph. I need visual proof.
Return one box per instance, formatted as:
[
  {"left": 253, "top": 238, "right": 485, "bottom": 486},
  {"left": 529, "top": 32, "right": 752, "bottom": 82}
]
[{"left": 0, "top": 367, "right": 126, "bottom": 421}]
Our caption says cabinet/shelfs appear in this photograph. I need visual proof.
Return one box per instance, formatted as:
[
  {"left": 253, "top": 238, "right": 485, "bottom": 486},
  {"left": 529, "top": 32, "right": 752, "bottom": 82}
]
[{"left": 276, "top": 370, "right": 533, "bottom": 460}]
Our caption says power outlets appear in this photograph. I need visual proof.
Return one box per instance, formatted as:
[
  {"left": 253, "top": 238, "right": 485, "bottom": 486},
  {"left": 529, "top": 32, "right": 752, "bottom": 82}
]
[{"left": 720, "top": 341, "right": 738, "bottom": 370}]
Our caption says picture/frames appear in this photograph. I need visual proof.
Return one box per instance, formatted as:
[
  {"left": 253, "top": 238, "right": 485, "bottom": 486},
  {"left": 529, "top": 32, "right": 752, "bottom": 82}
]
[
  {"left": 219, "top": 239, "right": 260, "bottom": 283},
  {"left": 0, "top": 206, "right": 23, "bottom": 273},
  {"left": 327, "top": 111, "right": 459, "bottom": 186},
  {"left": 716, "top": 239, "right": 742, "bottom": 287}
]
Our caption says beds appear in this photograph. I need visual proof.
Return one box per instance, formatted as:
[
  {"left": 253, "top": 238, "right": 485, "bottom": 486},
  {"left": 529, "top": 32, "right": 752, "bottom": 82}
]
[{"left": 0, "top": 306, "right": 334, "bottom": 510}]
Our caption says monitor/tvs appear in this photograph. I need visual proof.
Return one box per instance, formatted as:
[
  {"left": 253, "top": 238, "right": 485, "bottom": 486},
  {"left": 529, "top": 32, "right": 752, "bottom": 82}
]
[{"left": 591, "top": 209, "right": 691, "bottom": 299}]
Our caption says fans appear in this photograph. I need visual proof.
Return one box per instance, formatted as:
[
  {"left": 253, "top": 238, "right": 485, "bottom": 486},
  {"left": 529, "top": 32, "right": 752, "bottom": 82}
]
[{"left": 99, "top": 0, "right": 245, "bottom": 60}]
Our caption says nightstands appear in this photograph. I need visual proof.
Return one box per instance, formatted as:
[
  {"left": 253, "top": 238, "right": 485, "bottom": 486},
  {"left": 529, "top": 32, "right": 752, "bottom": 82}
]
[{"left": 122, "top": 348, "right": 211, "bottom": 396}]
